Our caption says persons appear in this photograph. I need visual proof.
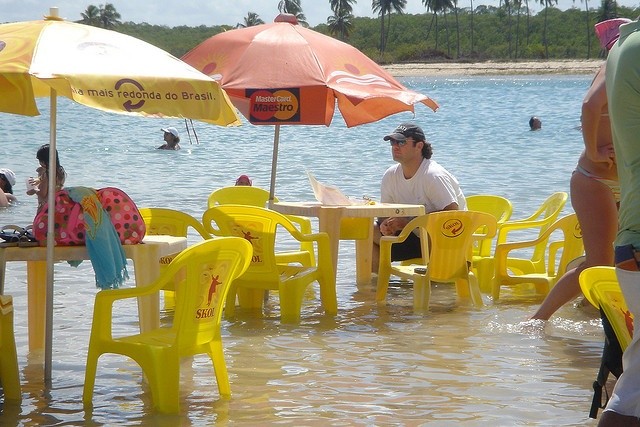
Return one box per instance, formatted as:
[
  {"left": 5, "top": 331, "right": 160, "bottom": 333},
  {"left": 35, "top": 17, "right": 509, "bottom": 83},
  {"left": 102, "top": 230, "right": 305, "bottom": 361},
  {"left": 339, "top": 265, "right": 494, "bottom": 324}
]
[
  {"left": 529, "top": 116, "right": 542, "bottom": 131},
  {"left": 0, "top": 167, "right": 16, "bottom": 206},
  {"left": 26, "top": 142, "right": 66, "bottom": 216},
  {"left": 599, "top": 13, "right": 640, "bottom": 425},
  {"left": 526, "top": 17, "right": 635, "bottom": 325},
  {"left": 156, "top": 127, "right": 181, "bottom": 151},
  {"left": 371, "top": 122, "right": 468, "bottom": 274}
]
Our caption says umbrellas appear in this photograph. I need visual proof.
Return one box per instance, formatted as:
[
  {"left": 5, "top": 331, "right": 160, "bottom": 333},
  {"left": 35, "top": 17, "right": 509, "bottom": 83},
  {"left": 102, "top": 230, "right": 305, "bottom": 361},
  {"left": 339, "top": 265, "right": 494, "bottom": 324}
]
[
  {"left": 1, "top": 5, "right": 243, "bottom": 385},
  {"left": 175, "top": 14, "right": 441, "bottom": 203}
]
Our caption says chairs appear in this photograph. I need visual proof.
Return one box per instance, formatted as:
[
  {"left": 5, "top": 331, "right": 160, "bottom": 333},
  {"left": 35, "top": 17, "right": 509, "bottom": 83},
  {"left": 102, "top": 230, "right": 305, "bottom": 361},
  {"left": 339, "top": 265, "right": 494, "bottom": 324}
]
[
  {"left": 81, "top": 236, "right": 253, "bottom": 412},
  {"left": 1, "top": 293, "right": 22, "bottom": 401},
  {"left": 375, "top": 210, "right": 498, "bottom": 310},
  {"left": 203, "top": 203, "right": 339, "bottom": 323},
  {"left": 208, "top": 186, "right": 314, "bottom": 274},
  {"left": 464, "top": 195, "right": 511, "bottom": 276},
  {"left": 477, "top": 191, "right": 569, "bottom": 294},
  {"left": 492, "top": 212, "right": 586, "bottom": 300},
  {"left": 139, "top": 206, "right": 216, "bottom": 306},
  {"left": 579, "top": 267, "right": 636, "bottom": 352}
]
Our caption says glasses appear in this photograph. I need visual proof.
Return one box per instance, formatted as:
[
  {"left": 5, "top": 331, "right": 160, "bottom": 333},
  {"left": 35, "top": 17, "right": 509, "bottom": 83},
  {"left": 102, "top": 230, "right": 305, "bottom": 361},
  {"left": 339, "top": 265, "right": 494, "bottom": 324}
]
[{"left": 389, "top": 140, "right": 415, "bottom": 146}]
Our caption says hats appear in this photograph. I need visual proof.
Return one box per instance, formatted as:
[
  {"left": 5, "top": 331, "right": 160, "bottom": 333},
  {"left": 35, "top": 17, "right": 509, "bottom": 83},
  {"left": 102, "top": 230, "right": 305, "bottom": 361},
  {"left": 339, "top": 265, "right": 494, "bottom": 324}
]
[
  {"left": 0, "top": 168, "right": 16, "bottom": 187},
  {"left": 161, "top": 127, "right": 179, "bottom": 138},
  {"left": 383, "top": 124, "right": 426, "bottom": 142}
]
